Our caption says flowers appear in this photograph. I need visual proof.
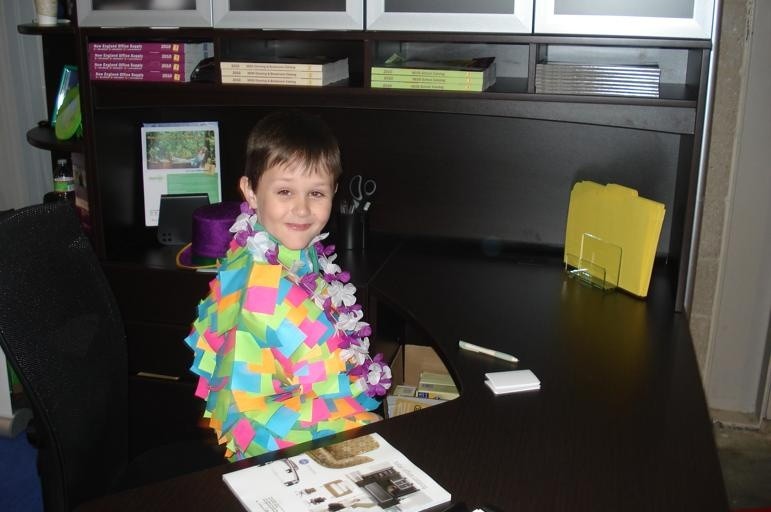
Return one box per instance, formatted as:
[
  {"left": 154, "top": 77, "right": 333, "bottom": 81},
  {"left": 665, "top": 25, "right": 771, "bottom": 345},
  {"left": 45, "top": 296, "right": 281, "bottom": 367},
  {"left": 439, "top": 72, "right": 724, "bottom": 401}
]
[{"left": 229, "top": 200, "right": 391, "bottom": 397}]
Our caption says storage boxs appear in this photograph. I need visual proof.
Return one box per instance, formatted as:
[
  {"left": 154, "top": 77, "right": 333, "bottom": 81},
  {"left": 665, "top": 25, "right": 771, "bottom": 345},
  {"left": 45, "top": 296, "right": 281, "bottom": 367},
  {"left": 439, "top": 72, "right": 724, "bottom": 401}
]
[{"left": 382, "top": 343, "right": 460, "bottom": 422}]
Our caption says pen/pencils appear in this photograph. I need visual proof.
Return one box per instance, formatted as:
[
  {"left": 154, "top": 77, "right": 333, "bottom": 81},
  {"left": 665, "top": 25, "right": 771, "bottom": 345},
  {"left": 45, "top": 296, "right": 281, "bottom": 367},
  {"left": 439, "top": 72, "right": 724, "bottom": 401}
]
[
  {"left": 339, "top": 198, "right": 371, "bottom": 214},
  {"left": 458, "top": 339, "right": 520, "bottom": 364}
]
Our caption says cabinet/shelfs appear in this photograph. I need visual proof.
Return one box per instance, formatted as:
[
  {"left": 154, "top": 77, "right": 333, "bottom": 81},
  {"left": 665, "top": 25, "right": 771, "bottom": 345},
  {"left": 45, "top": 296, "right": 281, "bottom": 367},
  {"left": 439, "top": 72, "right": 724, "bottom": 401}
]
[{"left": 13, "top": 23, "right": 712, "bottom": 259}]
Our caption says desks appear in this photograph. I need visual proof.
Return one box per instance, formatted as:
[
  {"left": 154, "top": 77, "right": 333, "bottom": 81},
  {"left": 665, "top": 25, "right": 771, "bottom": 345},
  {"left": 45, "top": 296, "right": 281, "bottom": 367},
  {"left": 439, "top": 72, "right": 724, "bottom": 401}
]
[{"left": 48, "top": 253, "right": 729, "bottom": 511}]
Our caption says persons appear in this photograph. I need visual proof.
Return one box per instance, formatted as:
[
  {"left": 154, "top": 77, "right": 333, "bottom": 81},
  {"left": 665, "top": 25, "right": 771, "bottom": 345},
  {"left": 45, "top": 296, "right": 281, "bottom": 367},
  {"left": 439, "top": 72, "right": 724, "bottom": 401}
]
[{"left": 184, "top": 109, "right": 393, "bottom": 463}]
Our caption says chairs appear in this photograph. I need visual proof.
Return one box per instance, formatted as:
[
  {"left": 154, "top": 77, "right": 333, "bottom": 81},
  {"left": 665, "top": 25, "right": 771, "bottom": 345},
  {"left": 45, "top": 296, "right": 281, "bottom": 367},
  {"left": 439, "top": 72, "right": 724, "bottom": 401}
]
[{"left": 2, "top": 201, "right": 222, "bottom": 511}]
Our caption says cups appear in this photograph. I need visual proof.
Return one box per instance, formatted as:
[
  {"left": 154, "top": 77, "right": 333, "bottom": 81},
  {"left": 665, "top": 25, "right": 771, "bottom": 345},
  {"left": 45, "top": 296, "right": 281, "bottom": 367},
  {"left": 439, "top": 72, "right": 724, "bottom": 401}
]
[
  {"left": 333, "top": 215, "right": 358, "bottom": 249},
  {"left": 34, "top": 0, "right": 58, "bottom": 27}
]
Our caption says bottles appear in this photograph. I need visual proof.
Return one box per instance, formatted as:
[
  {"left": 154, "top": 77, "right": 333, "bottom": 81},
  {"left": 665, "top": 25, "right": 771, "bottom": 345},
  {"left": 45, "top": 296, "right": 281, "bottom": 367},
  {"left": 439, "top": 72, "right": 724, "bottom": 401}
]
[{"left": 53, "top": 158, "right": 74, "bottom": 206}]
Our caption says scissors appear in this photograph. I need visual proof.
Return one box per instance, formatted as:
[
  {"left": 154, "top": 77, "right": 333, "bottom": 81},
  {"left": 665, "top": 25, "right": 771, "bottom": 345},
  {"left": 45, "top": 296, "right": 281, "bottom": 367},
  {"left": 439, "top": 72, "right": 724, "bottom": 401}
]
[{"left": 347, "top": 174, "right": 379, "bottom": 215}]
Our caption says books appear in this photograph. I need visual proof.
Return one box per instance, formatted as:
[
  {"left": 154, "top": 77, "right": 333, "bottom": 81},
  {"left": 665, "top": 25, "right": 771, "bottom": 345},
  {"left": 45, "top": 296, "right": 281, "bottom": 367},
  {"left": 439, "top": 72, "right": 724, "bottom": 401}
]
[
  {"left": 534, "top": 58, "right": 660, "bottom": 98},
  {"left": 84, "top": 39, "right": 217, "bottom": 84},
  {"left": 386, "top": 395, "right": 447, "bottom": 419},
  {"left": 220, "top": 57, "right": 352, "bottom": 90},
  {"left": 370, "top": 53, "right": 498, "bottom": 92}
]
